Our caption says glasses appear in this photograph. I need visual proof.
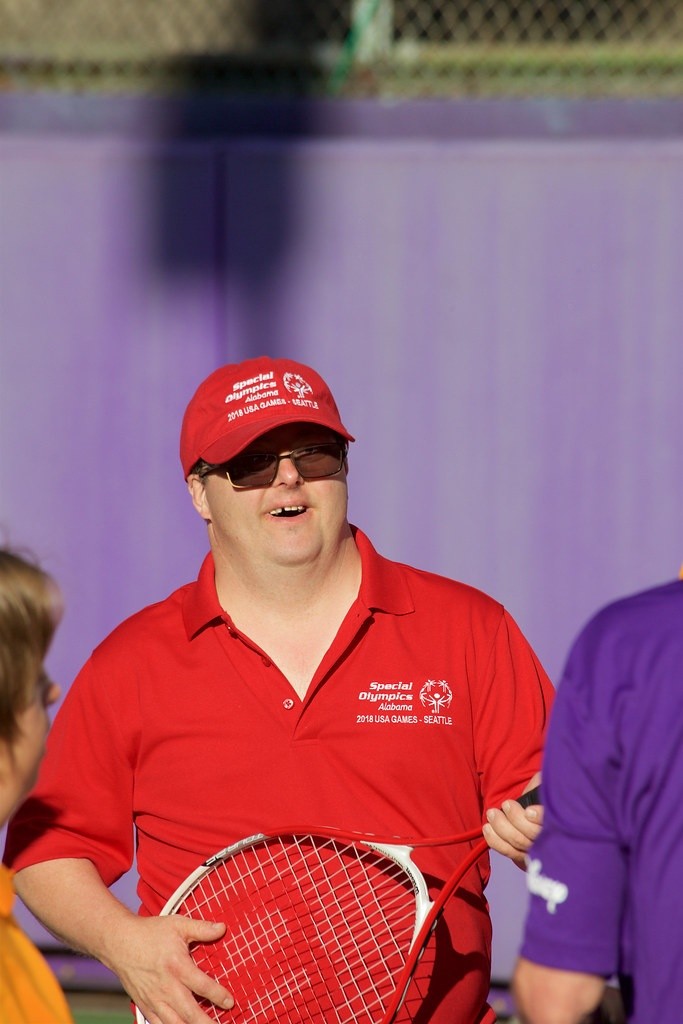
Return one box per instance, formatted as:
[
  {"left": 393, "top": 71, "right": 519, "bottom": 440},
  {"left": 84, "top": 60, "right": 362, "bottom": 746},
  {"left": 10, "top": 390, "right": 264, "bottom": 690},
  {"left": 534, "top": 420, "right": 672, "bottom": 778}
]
[{"left": 199, "top": 441, "right": 342, "bottom": 489}]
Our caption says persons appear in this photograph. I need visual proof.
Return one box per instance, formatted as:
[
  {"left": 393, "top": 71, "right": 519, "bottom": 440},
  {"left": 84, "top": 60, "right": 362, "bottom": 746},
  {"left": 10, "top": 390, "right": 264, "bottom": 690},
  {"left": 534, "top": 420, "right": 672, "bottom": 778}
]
[
  {"left": 0, "top": 548, "right": 73, "bottom": 1024},
  {"left": 513, "top": 579, "right": 683, "bottom": 1024},
  {"left": 0, "top": 356, "right": 558, "bottom": 1024}
]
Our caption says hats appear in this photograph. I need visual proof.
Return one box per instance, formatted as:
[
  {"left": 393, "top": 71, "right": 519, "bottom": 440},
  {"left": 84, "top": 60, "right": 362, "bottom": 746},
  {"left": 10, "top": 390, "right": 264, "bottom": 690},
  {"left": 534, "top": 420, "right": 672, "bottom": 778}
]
[{"left": 179, "top": 356, "right": 356, "bottom": 483}]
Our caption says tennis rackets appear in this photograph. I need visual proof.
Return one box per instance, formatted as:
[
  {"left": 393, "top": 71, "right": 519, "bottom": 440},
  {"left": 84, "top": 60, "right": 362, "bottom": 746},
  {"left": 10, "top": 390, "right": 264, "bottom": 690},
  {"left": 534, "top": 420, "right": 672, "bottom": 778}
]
[{"left": 134, "top": 786, "right": 540, "bottom": 1024}]
